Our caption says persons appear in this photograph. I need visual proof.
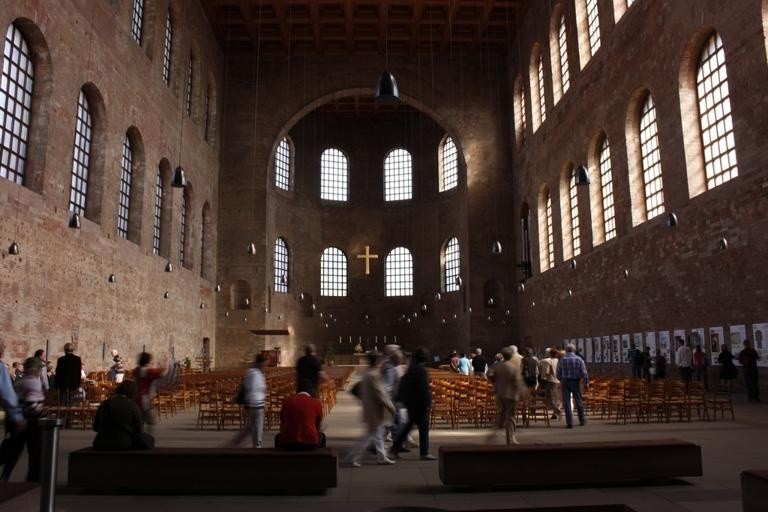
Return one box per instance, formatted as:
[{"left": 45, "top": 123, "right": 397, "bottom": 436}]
[
  {"left": 0, "top": 338, "right": 182, "bottom": 482},
  {"left": 295, "top": 343, "right": 329, "bottom": 399},
  {"left": 344, "top": 345, "right": 439, "bottom": 467},
  {"left": 718, "top": 344, "right": 739, "bottom": 383},
  {"left": 450, "top": 343, "right": 590, "bottom": 444},
  {"left": 739, "top": 339, "right": 761, "bottom": 404},
  {"left": 628, "top": 340, "right": 708, "bottom": 392},
  {"left": 216, "top": 354, "right": 270, "bottom": 448},
  {"left": 274, "top": 386, "right": 326, "bottom": 450}
]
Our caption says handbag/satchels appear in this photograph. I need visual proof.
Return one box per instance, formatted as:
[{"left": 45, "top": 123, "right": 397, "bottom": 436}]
[{"left": 349, "top": 381, "right": 362, "bottom": 399}]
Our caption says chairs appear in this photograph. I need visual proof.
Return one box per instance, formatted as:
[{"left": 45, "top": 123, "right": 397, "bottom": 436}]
[{"left": 35, "top": 359, "right": 734, "bottom": 431}]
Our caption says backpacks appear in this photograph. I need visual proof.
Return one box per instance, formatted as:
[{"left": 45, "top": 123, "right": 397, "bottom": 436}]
[{"left": 235, "top": 382, "right": 249, "bottom": 405}]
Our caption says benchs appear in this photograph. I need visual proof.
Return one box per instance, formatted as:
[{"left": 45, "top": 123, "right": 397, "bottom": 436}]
[
  {"left": 437, "top": 439, "right": 702, "bottom": 488},
  {"left": 65, "top": 448, "right": 339, "bottom": 496}
]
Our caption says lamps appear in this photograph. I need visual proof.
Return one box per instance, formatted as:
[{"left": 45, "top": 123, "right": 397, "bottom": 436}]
[{"left": 1, "top": 0, "right": 731, "bottom": 333}]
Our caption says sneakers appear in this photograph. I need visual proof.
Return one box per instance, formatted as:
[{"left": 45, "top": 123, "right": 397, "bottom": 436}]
[
  {"left": 340, "top": 435, "right": 437, "bottom": 468},
  {"left": 550, "top": 411, "right": 589, "bottom": 429}
]
